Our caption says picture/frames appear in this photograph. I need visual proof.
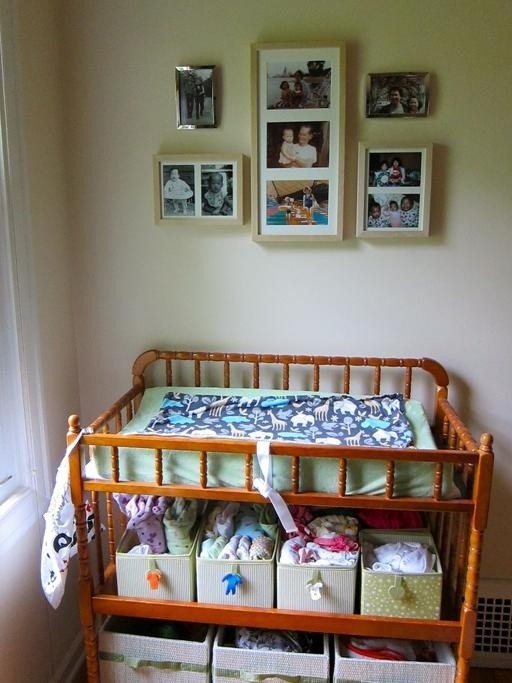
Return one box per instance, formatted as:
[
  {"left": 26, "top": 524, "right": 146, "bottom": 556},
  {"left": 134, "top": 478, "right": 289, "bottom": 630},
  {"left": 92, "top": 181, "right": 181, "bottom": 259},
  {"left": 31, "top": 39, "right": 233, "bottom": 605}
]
[
  {"left": 363, "top": 70, "right": 434, "bottom": 118},
  {"left": 357, "top": 141, "right": 431, "bottom": 237},
  {"left": 152, "top": 154, "right": 243, "bottom": 226},
  {"left": 175, "top": 63, "right": 216, "bottom": 128},
  {"left": 252, "top": 41, "right": 345, "bottom": 241}
]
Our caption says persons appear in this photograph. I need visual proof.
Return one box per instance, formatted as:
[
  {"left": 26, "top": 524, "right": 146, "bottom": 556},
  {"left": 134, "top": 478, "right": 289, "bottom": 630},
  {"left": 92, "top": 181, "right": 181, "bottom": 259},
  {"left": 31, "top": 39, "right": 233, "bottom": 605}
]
[
  {"left": 381, "top": 87, "right": 409, "bottom": 114},
  {"left": 295, "top": 125, "right": 317, "bottom": 167},
  {"left": 185, "top": 74, "right": 196, "bottom": 119},
  {"left": 400, "top": 197, "right": 417, "bottom": 227},
  {"left": 163, "top": 168, "right": 193, "bottom": 216},
  {"left": 389, "top": 168, "right": 401, "bottom": 187},
  {"left": 384, "top": 201, "right": 401, "bottom": 227},
  {"left": 303, "top": 186, "right": 314, "bottom": 224},
  {"left": 407, "top": 95, "right": 419, "bottom": 114},
  {"left": 278, "top": 128, "right": 297, "bottom": 168},
  {"left": 285, "top": 197, "right": 291, "bottom": 222},
  {"left": 195, "top": 79, "right": 205, "bottom": 119},
  {"left": 373, "top": 161, "right": 390, "bottom": 187},
  {"left": 280, "top": 81, "right": 291, "bottom": 102},
  {"left": 369, "top": 202, "right": 384, "bottom": 227},
  {"left": 389, "top": 160, "right": 406, "bottom": 185},
  {"left": 292, "top": 82, "right": 303, "bottom": 107},
  {"left": 204, "top": 173, "right": 230, "bottom": 216},
  {"left": 294, "top": 70, "right": 308, "bottom": 98}
]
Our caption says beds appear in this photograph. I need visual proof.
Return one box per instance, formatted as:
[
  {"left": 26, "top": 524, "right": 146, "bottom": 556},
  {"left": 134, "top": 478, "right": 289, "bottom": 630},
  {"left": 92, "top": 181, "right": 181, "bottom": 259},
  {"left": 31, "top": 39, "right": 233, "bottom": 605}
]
[{"left": 67, "top": 350, "right": 494, "bottom": 683}]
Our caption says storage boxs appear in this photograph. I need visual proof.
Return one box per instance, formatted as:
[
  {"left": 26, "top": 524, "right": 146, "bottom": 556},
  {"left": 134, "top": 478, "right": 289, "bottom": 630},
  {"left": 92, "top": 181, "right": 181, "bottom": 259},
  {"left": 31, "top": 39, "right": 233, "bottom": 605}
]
[
  {"left": 360, "top": 528, "right": 442, "bottom": 619},
  {"left": 98, "top": 616, "right": 211, "bottom": 683},
  {"left": 276, "top": 535, "right": 360, "bottom": 615},
  {"left": 212, "top": 627, "right": 333, "bottom": 683},
  {"left": 196, "top": 530, "right": 278, "bottom": 609},
  {"left": 116, "top": 525, "right": 199, "bottom": 601},
  {"left": 333, "top": 635, "right": 456, "bottom": 683}
]
